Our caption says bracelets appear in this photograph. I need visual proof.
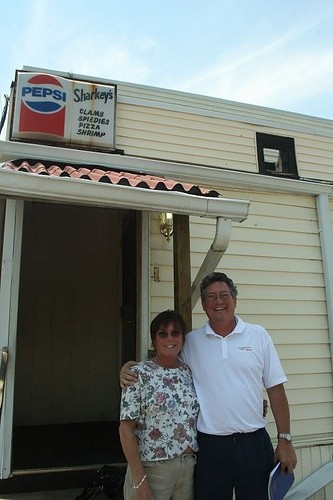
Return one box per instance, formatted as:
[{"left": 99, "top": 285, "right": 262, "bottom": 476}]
[{"left": 134, "top": 474, "right": 146, "bottom": 488}]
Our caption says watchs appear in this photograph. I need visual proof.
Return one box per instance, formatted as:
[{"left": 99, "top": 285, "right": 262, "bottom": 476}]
[{"left": 278, "top": 433, "right": 292, "bottom": 441}]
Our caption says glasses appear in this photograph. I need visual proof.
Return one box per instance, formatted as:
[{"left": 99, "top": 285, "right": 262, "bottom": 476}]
[
  {"left": 155, "top": 330, "right": 182, "bottom": 338},
  {"left": 203, "top": 293, "right": 233, "bottom": 301}
]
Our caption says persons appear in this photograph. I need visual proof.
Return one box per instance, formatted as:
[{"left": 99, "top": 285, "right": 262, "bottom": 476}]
[
  {"left": 120, "top": 272, "right": 297, "bottom": 500},
  {"left": 119, "top": 310, "right": 268, "bottom": 500}
]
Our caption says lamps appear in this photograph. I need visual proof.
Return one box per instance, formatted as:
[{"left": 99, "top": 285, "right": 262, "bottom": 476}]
[{"left": 160, "top": 213, "right": 173, "bottom": 243}]
